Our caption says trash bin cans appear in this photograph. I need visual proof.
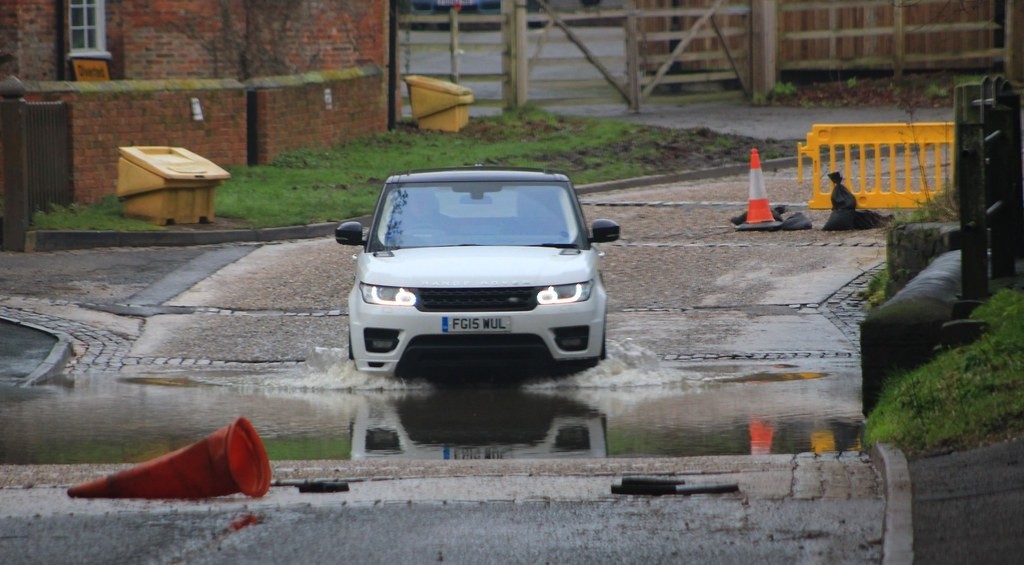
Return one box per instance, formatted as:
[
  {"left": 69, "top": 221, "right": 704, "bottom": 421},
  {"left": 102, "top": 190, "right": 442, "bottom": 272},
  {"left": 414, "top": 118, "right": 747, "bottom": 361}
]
[
  {"left": 114, "top": 144, "right": 230, "bottom": 224},
  {"left": 405, "top": 74, "right": 472, "bottom": 132}
]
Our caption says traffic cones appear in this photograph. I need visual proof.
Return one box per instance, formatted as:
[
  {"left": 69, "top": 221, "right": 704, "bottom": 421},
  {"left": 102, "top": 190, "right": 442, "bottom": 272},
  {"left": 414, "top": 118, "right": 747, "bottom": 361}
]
[
  {"left": 66, "top": 416, "right": 274, "bottom": 501},
  {"left": 734, "top": 146, "right": 783, "bottom": 232}
]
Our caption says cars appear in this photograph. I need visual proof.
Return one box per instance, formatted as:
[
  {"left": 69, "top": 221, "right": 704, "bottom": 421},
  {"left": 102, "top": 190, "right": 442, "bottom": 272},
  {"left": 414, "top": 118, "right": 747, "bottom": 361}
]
[{"left": 334, "top": 166, "right": 621, "bottom": 377}]
